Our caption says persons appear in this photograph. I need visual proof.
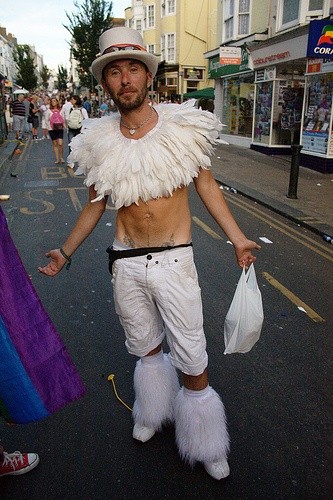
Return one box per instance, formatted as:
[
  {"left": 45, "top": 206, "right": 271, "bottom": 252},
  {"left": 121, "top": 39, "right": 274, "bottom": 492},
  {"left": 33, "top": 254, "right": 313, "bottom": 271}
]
[
  {"left": 0, "top": 81, "right": 181, "bottom": 144},
  {"left": 45, "top": 98, "right": 66, "bottom": 163},
  {"left": 38, "top": 27, "right": 262, "bottom": 479},
  {"left": 67, "top": 95, "right": 89, "bottom": 154},
  {"left": 0, "top": 207, "right": 87, "bottom": 477}
]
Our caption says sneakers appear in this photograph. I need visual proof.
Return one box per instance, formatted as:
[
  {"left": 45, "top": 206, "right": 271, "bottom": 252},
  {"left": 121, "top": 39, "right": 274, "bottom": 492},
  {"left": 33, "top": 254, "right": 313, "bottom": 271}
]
[{"left": 0, "top": 448, "right": 41, "bottom": 477}]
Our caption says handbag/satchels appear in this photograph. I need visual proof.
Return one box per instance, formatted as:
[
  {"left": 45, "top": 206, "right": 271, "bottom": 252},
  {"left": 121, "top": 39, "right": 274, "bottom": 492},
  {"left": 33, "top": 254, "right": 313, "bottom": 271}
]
[{"left": 221, "top": 260, "right": 265, "bottom": 356}]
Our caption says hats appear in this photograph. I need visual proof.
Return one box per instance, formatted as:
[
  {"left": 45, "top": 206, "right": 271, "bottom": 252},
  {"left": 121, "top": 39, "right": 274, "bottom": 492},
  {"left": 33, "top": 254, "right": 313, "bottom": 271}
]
[{"left": 91, "top": 25, "right": 160, "bottom": 86}]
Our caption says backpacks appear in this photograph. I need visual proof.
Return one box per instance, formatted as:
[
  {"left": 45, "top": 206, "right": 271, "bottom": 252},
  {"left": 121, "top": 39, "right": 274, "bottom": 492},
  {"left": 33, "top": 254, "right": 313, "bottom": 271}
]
[
  {"left": 50, "top": 109, "right": 64, "bottom": 131},
  {"left": 67, "top": 107, "right": 82, "bottom": 130}
]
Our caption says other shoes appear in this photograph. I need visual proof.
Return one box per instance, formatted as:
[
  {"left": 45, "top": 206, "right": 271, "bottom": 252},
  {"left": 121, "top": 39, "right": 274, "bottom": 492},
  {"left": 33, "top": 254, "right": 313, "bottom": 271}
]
[
  {"left": 174, "top": 387, "right": 232, "bottom": 482},
  {"left": 131, "top": 357, "right": 181, "bottom": 445},
  {"left": 33, "top": 135, "right": 51, "bottom": 141},
  {"left": 60, "top": 158, "right": 65, "bottom": 163},
  {"left": 54, "top": 158, "right": 60, "bottom": 164}
]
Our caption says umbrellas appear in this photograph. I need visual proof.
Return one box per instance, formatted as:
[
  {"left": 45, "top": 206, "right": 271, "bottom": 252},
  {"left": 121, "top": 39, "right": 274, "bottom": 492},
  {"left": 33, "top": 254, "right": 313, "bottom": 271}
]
[{"left": 183, "top": 87, "right": 214, "bottom": 100}]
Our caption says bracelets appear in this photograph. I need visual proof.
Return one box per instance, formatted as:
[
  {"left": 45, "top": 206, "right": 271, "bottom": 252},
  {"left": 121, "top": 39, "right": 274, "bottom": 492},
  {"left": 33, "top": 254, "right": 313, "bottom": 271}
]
[{"left": 60, "top": 246, "right": 72, "bottom": 262}]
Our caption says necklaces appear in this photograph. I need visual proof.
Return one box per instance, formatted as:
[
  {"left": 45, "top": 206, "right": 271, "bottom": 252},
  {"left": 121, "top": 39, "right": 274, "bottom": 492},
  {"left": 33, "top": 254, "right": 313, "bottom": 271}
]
[{"left": 122, "top": 108, "right": 152, "bottom": 135}]
